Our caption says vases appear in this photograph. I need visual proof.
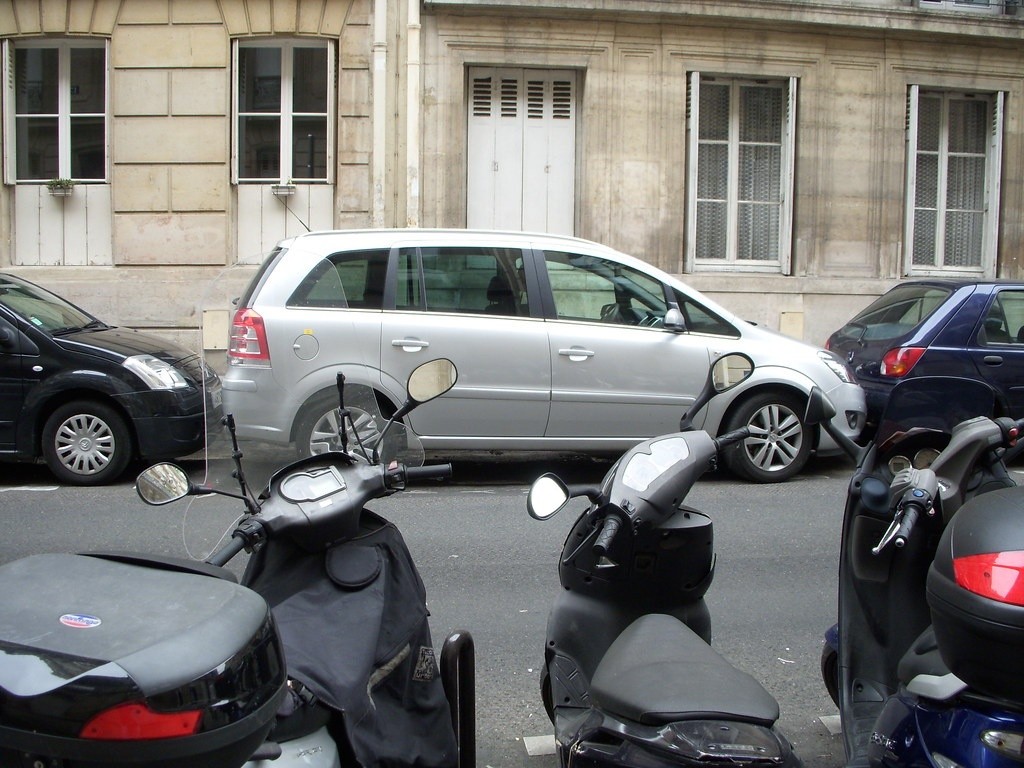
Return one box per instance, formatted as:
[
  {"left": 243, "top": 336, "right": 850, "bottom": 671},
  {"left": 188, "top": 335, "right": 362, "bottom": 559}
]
[{"left": 271, "top": 184, "right": 295, "bottom": 195}]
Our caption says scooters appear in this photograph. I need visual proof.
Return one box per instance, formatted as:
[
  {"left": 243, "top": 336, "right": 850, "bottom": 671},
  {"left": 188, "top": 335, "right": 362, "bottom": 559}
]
[
  {"left": 0, "top": 358, "right": 480, "bottom": 768},
  {"left": 802, "top": 376, "right": 1024, "bottom": 768},
  {"left": 527, "top": 351, "right": 803, "bottom": 767}
]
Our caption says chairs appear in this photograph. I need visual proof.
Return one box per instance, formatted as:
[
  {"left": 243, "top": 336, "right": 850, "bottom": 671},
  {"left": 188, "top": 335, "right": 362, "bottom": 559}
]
[
  {"left": 984, "top": 305, "right": 1011, "bottom": 342},
  {"left": 483, "top": 276, "right": 513, "bottom": 314}
]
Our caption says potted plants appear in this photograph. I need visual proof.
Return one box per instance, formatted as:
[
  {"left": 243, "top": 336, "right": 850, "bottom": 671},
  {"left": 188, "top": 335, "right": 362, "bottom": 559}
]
[{"left": 47, "top": 178, "right": 74, "bottom": 196}]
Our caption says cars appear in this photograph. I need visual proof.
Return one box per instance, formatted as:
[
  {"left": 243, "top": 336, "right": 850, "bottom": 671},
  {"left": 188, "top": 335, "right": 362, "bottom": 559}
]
[
  {"left": 216, "top": 228, "right": 869, "bottom": 484},
  {"left": 0, "top": 274, "right": 225, "bottom": 489},
  {"left": 827, "top": 276, "right": 1024, "bottom": 466}
]
